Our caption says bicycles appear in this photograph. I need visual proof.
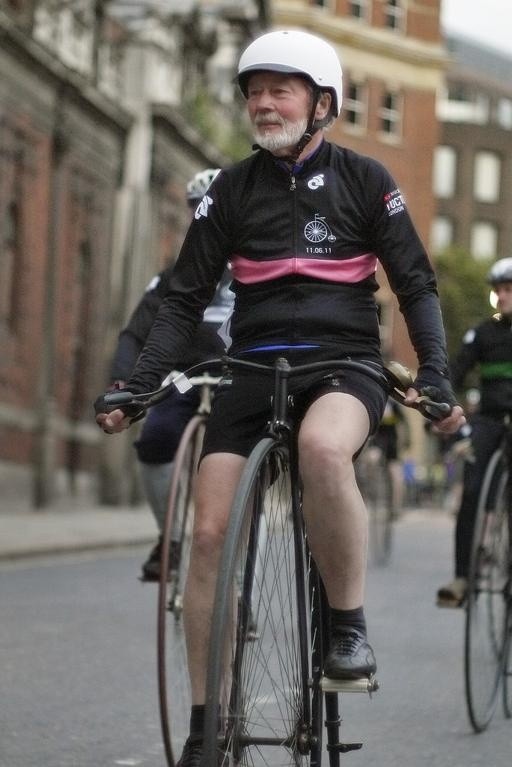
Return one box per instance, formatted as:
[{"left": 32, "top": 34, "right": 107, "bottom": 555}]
[{"left": 424, "top": 411, "right": 512, "bottom": 734}]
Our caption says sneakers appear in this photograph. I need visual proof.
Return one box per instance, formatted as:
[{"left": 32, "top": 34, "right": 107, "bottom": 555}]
[
  {"left": 437, "top": 578, "right": 467, "bottom": 602},
  {"left": 175, "top": 734, "right": 211, "bottom": 766},
  {"left": 324, "top": 625, "right": 376, "bottom": 677},
  {"left": 141, "top": 538, "right": 180, "bottom": 579}
]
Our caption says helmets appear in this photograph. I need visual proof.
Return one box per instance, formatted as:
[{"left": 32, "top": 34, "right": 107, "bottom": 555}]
[
  {"left": 490, "top": 257, "right": 512, "bottom": 283},
  {"left": 233, "top": 29, "right": 343, "bottom": 117},
  {"left": 186, "top": 167, "right": 221, "bottom": 201}
]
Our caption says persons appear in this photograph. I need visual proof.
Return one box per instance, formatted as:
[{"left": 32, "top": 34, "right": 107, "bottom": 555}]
[
  {"left": 365, "top": 398, "right": 410, "bottom": 521},
  {"left": 107, "top": 165, "right": 285, "bottom": 639},
  {"left": 92, "top": 28, "right": 467, "bottom": 767},
  {"left": 435, "top": 259, "right": 511, "bottom": 607}
]
